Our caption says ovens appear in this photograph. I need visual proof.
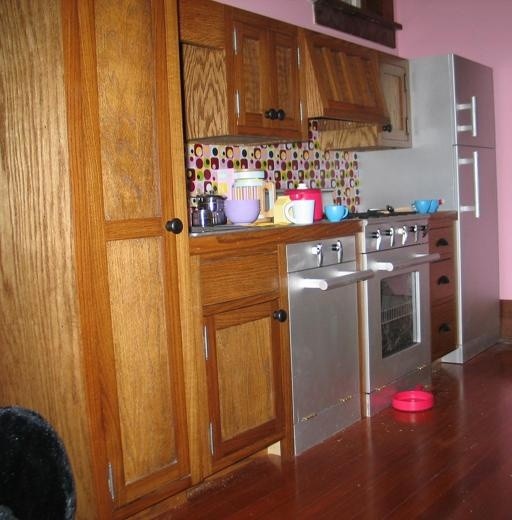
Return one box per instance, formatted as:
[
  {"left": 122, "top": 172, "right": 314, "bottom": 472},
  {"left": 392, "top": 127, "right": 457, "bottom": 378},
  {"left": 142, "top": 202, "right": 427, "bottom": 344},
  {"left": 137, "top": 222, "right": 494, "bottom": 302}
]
[{"left": 285, "top": 235, "right": 364, "bottom": 457}]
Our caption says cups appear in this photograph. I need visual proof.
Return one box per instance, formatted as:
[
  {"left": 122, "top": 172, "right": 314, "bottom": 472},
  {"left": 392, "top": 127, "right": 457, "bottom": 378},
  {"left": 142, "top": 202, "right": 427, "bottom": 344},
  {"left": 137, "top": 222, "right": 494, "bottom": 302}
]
[
  {"left": 414, "top": 199, "right": 440, "bottom": 213},
  {"left": 274, "top": 189, "right": 323, "bottom": 226},
  {"left": 325, "top": 206, "right": 348, "bottom": 223},
  {"left": 232, "top": 170, "right": 275, "bottom": 226}
]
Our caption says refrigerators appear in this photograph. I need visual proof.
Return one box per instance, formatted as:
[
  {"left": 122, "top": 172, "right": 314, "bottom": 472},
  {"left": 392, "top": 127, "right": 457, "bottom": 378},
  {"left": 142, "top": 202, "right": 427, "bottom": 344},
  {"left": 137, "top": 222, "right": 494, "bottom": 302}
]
[{"left": 359, "top": 54, "right": 501, "bottom": 366}]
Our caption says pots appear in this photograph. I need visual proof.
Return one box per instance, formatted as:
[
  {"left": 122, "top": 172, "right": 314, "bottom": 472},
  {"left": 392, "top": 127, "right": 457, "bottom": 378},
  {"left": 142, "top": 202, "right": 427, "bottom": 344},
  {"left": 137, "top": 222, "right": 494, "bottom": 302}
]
[{"left": 192, "top": 191, "right": 227, "bottom": 229}]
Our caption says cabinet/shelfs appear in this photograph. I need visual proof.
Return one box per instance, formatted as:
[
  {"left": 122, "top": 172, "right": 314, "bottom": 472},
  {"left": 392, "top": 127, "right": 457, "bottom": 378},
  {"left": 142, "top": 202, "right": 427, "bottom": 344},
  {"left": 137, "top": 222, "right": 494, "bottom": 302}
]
[
  {"left": 429, "top": 209, "right": 459, "bottom": 365},
  {"left": 180, "top": 5, "right": 301, "bottom": 144},
  {"left": 0, "top": 0, "right": 192, "bottom": 520},
  {"left": 190, "top": 230, "right": 294, "bottom": 504},
  {"left": 321, "top": 50, "right": 412, "bottom": 152}
]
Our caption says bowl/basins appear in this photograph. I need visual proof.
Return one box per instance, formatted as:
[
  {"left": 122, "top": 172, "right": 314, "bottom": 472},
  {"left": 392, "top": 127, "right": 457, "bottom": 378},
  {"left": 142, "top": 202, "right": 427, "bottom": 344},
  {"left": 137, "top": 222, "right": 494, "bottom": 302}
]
[
  {"left": 391, "top": 389, "right": 435, "bottom": 413},
  {"left": 224, "top": 200, "right": 260, "bottom": 226}
]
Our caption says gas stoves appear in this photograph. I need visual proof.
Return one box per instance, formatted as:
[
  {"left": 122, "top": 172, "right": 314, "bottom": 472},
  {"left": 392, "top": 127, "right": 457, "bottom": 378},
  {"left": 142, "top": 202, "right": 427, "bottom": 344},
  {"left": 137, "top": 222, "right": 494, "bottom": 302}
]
[{"left": 322, "top": 204, "right": 420, "bottom": 221}]
